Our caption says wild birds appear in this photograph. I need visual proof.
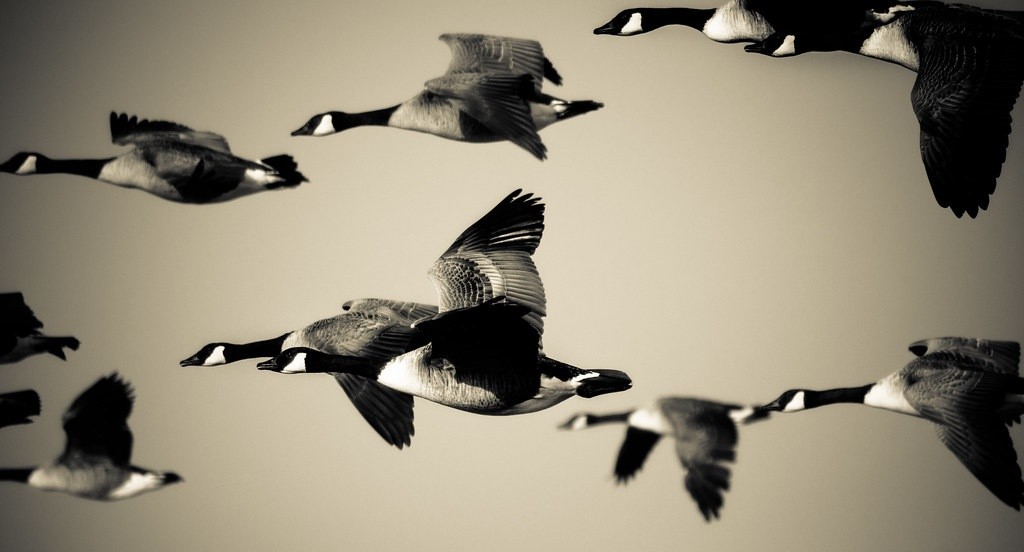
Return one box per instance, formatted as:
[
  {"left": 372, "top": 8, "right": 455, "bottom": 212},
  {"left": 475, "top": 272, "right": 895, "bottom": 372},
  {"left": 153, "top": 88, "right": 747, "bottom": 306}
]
[
  {"left": 177, "top": 188, "right": 634, "bottom": 453},
  {"left": 0, "top": 111, "right": 312, "bottom": 205},
  {"left": 290, "top": 31, "right": 605, "bottom": 162},
  {"left": 761, "top": 335, "right": 1023, "bottom": 515},
  {"left": 0, "top": 292, "right": 187, "bottom": 502},
  {"left": 594, "top": 1, "right": 1023, "bottom": 220},
  {"left": 556, "top": 396, "right": 770, "bottom": 522}
]
